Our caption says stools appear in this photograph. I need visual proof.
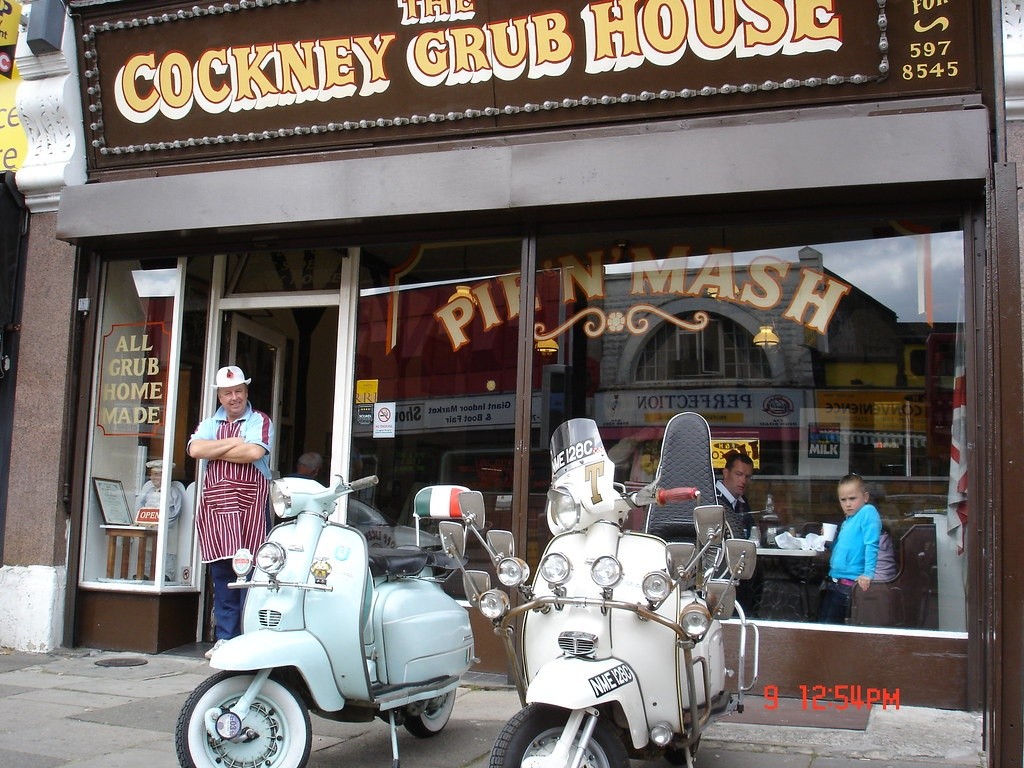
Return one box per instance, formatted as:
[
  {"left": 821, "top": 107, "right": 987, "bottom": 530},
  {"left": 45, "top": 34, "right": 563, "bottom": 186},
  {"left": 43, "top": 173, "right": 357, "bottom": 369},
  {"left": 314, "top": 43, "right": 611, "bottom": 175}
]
[{"left": 104, "top": 529, "right": 157, "bottom": 584}]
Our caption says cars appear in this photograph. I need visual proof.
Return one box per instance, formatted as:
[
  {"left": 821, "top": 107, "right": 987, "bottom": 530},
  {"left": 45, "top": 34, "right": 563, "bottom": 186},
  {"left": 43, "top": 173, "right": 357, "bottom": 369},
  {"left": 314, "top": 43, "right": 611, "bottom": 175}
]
[{"left": 347, "top": 495, "right": 448, "bottom": 578}]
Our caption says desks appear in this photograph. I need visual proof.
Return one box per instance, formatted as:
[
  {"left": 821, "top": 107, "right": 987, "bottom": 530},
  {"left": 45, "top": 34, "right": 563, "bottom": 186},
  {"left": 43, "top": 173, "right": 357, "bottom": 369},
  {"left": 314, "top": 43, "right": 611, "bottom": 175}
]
[{"left": 754, "top": 540, "right": 840, "bottom": 623}]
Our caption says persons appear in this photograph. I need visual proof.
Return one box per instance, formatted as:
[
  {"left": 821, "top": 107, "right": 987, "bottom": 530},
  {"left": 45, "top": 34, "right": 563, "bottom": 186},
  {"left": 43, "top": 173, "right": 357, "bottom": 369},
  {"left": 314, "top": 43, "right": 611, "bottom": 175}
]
[
  {"left": 714, "top": 453, "right": 755, "bottom": 540},
  {"left": 875, "top": 526, "right": 897, "bottom": 582},
  {"left": 132, "top": 459, "right": 184, "bottom": 581},
  {"left": 185, "top": 366, "right": 273, "bottom": 659},
  {"left": 283, "top": 452, "right": 324, "bottom": 481},
  {"left": 815, "top": 474, "right": 882, "bottom": 625}
]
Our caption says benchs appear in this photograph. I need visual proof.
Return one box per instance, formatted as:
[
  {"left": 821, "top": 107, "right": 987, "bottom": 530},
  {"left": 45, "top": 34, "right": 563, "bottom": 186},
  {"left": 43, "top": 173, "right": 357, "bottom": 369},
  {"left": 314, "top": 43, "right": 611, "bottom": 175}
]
[{"left": 844, "top": 519, "right": 942, "bottom": 630}]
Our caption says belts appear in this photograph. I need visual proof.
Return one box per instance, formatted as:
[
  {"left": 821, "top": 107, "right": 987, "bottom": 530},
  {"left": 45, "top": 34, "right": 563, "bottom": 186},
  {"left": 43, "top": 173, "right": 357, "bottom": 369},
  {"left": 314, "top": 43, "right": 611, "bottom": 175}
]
[{"left": 831, "top": 578, "right": 854, "bottom": 587}]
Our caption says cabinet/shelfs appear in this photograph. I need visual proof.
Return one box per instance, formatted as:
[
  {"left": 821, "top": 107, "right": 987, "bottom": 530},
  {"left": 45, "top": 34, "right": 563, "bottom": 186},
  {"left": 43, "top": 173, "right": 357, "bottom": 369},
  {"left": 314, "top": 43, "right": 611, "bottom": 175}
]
[{"left": 143, "top": 363, "right": 194, "bottom": 484}]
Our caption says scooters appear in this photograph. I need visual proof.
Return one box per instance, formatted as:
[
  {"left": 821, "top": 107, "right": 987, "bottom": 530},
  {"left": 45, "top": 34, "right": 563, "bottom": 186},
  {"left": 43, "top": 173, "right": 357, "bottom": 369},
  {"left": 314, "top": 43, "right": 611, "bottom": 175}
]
[
  {"left": 172, "top": 467, "right": 481, "bottom": 768},
  {"left": 436, "top": 410, "right": 762, "bottom": 768}
]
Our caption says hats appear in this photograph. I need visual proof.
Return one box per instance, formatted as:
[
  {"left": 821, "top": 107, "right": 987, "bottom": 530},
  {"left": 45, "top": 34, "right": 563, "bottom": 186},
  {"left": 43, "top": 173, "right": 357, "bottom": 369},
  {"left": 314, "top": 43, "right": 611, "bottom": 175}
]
[{"left": 209, "top": 366, "right": 252, "bottom": 390}]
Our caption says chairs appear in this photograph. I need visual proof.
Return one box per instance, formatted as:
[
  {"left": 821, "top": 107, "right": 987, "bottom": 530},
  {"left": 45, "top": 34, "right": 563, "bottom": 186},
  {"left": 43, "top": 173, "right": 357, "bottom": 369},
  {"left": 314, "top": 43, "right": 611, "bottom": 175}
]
[
  {"left": 412, "top": 484, "right": 473, "bottom": 553},
  {"left": 645, "top": 411, "right": 733, "bottom": 577}
]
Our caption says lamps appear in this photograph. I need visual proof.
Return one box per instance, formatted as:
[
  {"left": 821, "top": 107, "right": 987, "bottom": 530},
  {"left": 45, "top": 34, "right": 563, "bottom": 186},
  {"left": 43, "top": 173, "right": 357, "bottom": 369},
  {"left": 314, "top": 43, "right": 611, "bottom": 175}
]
[
  {"left": 753, "top": 326, "right": 780, "bottom": 350},
  {"left": 534, "top": 334, "right": 560, "bottom": 353}
]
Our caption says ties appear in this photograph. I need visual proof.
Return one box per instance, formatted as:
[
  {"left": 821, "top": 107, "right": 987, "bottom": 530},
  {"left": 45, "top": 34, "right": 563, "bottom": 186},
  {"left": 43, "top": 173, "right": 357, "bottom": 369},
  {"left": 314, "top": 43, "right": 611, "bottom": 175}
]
[{"left": 735, "top": 500, "right": 740, "bottom": 515}]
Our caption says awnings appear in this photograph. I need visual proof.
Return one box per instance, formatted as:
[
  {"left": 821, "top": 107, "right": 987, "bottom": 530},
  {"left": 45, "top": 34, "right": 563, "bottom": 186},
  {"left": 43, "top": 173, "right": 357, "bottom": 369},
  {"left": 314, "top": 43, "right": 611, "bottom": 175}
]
[{"left": 55, "top": 107, "right": 991, "bottom": 242}]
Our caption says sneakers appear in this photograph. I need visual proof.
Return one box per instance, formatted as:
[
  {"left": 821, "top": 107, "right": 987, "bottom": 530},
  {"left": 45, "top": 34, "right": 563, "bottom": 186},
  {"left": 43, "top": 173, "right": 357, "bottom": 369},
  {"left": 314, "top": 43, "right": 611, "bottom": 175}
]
[{"left": 205, "top": 639, "right": 230, "bottom": 659}]
